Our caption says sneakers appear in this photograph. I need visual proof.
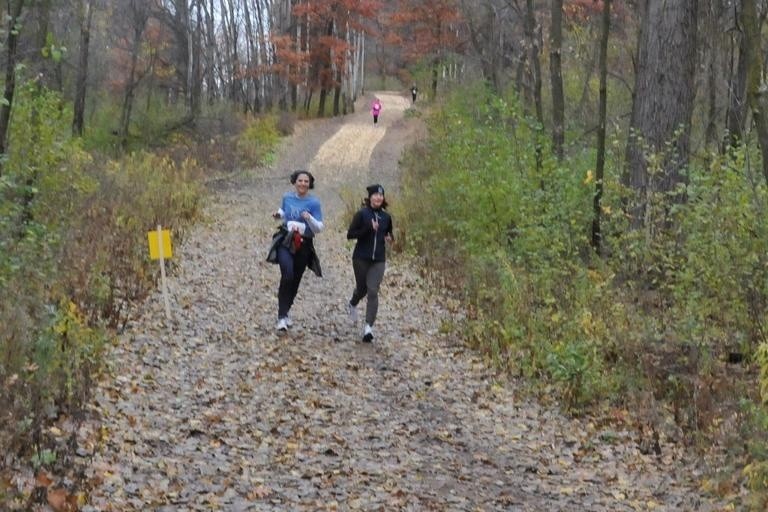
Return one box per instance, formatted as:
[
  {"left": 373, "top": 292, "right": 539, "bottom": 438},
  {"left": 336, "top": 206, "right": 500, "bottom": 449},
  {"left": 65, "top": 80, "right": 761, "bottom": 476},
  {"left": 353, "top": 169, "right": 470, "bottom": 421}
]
[
  {"left": 277, "top": 316, "right": 293, "bottom": 331},
  {"left": 363, "top": 321, "right": 374, "bottom": 342},
  {"left": 349, "top": 301, "right": 359, "bottom": 321}
]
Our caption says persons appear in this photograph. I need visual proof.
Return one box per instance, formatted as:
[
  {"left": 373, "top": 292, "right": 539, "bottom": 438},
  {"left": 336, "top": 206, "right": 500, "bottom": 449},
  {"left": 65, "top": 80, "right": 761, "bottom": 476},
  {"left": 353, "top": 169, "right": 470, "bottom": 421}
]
[
  {"left": 347, "top": 185, "right": 395, "bottom": 343},
  {"left": 266, "top": 169, "right": 324, "bottom": 332},
  {"left": 372, "top": 98, "right": 381, "bottom": 123},
  {"left": 410, "top": 82, "right": 419, "bottom": 102}
]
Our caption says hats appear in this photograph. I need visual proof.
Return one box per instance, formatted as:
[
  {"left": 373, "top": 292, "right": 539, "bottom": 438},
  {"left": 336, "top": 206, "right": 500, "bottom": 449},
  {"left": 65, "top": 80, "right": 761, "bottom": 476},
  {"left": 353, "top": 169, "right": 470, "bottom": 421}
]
[{"left": 367, "top": 184, "right": 384, "bottom": 194}]
